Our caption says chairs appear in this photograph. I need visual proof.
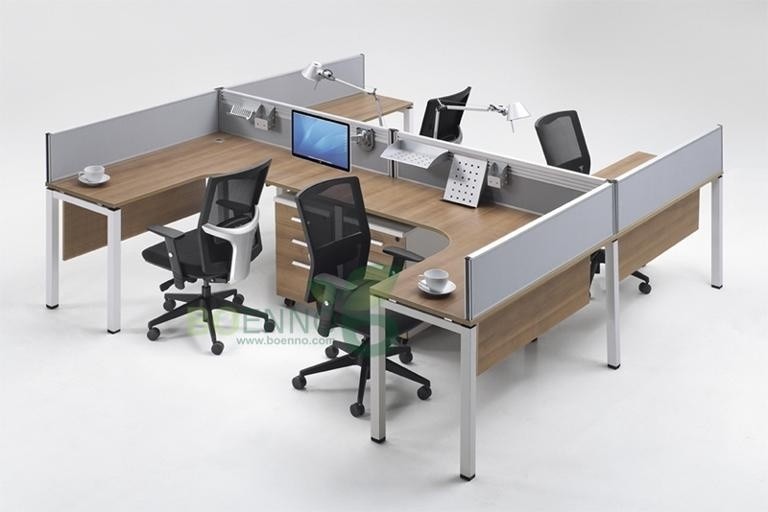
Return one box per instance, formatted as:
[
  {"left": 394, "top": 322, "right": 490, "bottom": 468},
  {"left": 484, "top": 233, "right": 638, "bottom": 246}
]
[
  {"left": 415, "top": 86, "right": 474, "bottom": 144},
  {"left": 293, "top": 179, "right": 433, "bottom": 418},
  {"left": 138, "top": 158, "right": 276, "bottom": 355},
  {"left": 533, "top": 110, "right": 654, "bottom": 295}
]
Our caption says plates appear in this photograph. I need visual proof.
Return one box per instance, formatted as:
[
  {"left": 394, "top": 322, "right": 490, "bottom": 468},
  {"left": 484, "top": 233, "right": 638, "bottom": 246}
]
[
  {"left": 416, "top": 281, "right": 456, "bottom": 297},
  {"left": 77, "top": 173, "right": 111, "bottom": 187}
]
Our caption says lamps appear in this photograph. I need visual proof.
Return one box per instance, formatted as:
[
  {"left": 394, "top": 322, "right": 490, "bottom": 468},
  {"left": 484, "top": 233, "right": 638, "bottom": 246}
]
[
  {"left": 429, "top": 98, "right": 530, "bottom": 145},
  {"left": 303, "top": 60, "right": 386, "bottom": 125}
]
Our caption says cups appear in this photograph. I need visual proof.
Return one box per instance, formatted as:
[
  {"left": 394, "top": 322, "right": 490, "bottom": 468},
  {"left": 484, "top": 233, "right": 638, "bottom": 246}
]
[
  {"left": 78, "top": 166, "right": 105, "bottom": 181},
  {"left": 417, "top": 268, "right": 450, "bottom": 292}
]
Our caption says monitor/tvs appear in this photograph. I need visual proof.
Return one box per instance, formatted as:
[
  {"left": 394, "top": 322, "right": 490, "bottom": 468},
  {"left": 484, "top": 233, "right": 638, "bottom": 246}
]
[{"left": 290, "top": 109, "right": 352, "bottom": 174}]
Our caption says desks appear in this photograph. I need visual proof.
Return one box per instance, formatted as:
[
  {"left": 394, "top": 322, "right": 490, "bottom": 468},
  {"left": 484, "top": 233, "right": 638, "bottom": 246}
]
[
  {"left": 583, "top": 149, "right": 656, "bottom": 180},
  {"left": 42, "top": 133, "right": 547, "bottom": 480},
  {"left": 295, "top": 89, "right": 412, "bottom": 128}
]
